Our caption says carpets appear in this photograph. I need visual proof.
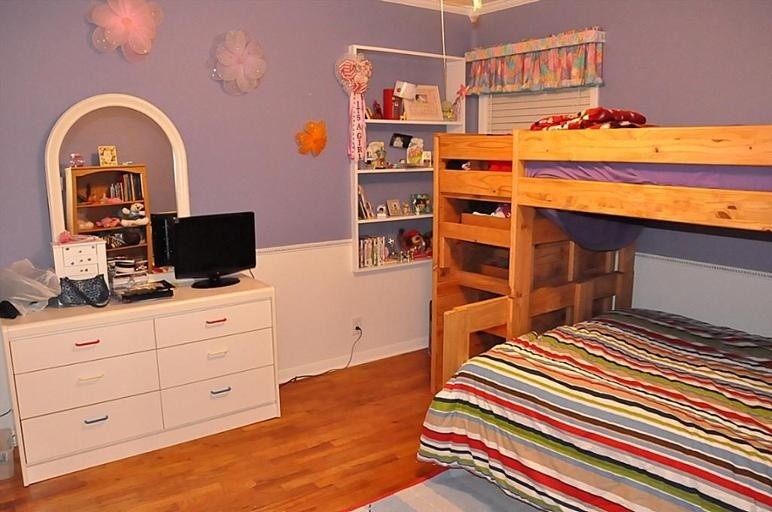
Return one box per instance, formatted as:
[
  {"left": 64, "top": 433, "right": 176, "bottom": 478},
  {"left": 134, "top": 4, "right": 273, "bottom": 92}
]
[{"left": 341, "top": 465, "right": 540, "bottom": 512}]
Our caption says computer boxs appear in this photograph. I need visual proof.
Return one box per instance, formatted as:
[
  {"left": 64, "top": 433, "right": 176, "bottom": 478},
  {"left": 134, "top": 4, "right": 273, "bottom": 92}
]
[{"left": 151, "top": 211, "right": 177, "bottom": 267}]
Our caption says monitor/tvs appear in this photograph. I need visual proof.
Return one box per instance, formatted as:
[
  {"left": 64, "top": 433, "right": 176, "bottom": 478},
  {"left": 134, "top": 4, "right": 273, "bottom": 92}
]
[{"left": 169, "top": 210, "right": 257, "bottom": 289}]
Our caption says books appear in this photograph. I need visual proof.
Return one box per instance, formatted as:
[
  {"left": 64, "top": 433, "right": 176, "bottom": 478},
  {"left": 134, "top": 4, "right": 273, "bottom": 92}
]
[
  {"left": 112, "top": 278, "right": 175, "bottom": 302},
  {"left": 106, "top": 253, "right": 148, "bottom": 278},
  {"left": 357, "top": 185, "right": 385, "bottom": 268},
  {"left": 109, "top": 173, "right": 143, "bottom": 202}
]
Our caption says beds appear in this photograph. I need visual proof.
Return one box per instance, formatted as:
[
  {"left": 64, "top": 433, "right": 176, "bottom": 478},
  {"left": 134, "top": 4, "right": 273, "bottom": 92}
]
[{"left": 429, "top": 125, "right": 772, "bottom": 511}]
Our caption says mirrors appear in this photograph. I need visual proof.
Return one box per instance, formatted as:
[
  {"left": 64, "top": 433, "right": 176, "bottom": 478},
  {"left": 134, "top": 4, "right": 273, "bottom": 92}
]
[{"left": 44, "top": 93, "right": 190, "bottom": 290}]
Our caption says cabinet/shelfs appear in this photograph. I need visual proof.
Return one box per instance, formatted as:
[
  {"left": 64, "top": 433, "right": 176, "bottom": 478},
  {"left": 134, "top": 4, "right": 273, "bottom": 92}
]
[
  {"left": 65, "top": 166, "right": 153, "bottom": 275},
  {"left": 50, "top": 241, "right": 111, "bottom": 305},
  {"left": 348, "top": 44, "right": 466, "bottom": 274},
  {"left": 0, "top": 286, "right": 281, "bottom": 487}
]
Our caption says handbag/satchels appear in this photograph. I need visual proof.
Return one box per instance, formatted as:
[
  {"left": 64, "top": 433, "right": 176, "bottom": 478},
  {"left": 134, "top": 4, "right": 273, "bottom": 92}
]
[{"left": 58, "top": 274, "right": 109, "bottom": 306}]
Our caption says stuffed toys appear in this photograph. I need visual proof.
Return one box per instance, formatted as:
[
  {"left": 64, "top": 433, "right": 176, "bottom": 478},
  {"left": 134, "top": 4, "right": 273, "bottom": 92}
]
[{"left": 75, "top": 200, "right": 149, "bottom": 248}]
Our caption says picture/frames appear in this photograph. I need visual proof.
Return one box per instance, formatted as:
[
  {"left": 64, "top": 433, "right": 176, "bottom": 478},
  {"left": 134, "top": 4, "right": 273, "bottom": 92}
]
[
  {"left": 386, "top": 193, "right": 432, "bottom": 216},
  {"left": 98, "top": 145, "right": 119, "bottom": 167}
]
